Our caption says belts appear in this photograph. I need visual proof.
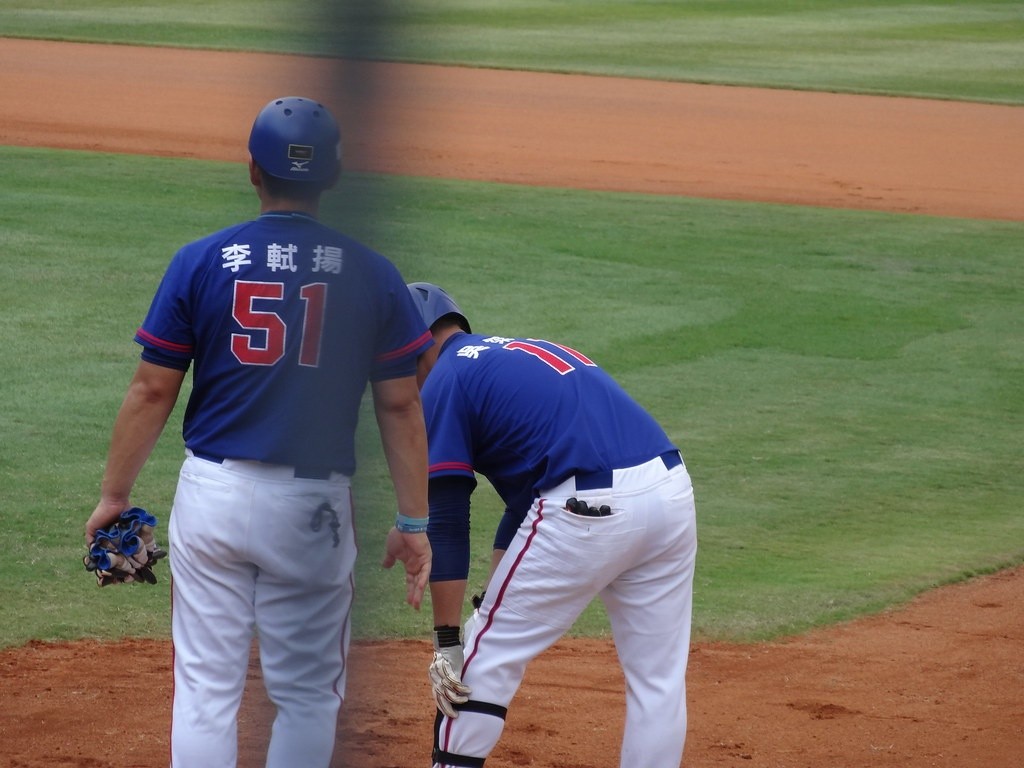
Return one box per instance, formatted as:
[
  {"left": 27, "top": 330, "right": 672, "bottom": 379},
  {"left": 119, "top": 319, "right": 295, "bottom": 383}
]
[
  {"left": 193, "top": 451, "right": 331, "bottom": 480},
  {"left": 575, "top": 450, "right": 682, "bottom": 491}
]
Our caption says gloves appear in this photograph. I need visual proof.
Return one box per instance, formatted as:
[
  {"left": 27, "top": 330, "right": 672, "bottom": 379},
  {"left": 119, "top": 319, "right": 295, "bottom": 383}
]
[
  {"left": 565, "top": 498, "right": 611, "bottom": 517},
  {"left": 82, "top": 507, "right": 168, "bottom": 588},
  {"left": 428, "top": 629, "right": 472, "bottom": 718}
]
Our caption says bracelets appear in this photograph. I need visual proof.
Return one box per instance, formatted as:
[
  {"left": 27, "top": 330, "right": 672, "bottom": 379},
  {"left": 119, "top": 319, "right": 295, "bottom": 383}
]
[{"left": 395, "top": 512, "right": 430, "bottom": 533}]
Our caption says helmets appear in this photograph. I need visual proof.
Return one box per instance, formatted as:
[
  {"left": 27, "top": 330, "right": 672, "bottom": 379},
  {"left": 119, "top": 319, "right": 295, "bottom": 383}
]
[
  {"left": 403, "top": 282, "right": 472, "bottom": 333},
  {"left": 248, "top": 97, "right": 342, "bottom": 183}
]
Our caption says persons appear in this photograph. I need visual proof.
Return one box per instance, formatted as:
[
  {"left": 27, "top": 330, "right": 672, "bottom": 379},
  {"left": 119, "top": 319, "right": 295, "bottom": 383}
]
[
  {"left": 85, "top": 96, "right": 432, "bottom": 768},
  {"left": 407, "top": 282, "right": 699, "bottom": 768}
]
